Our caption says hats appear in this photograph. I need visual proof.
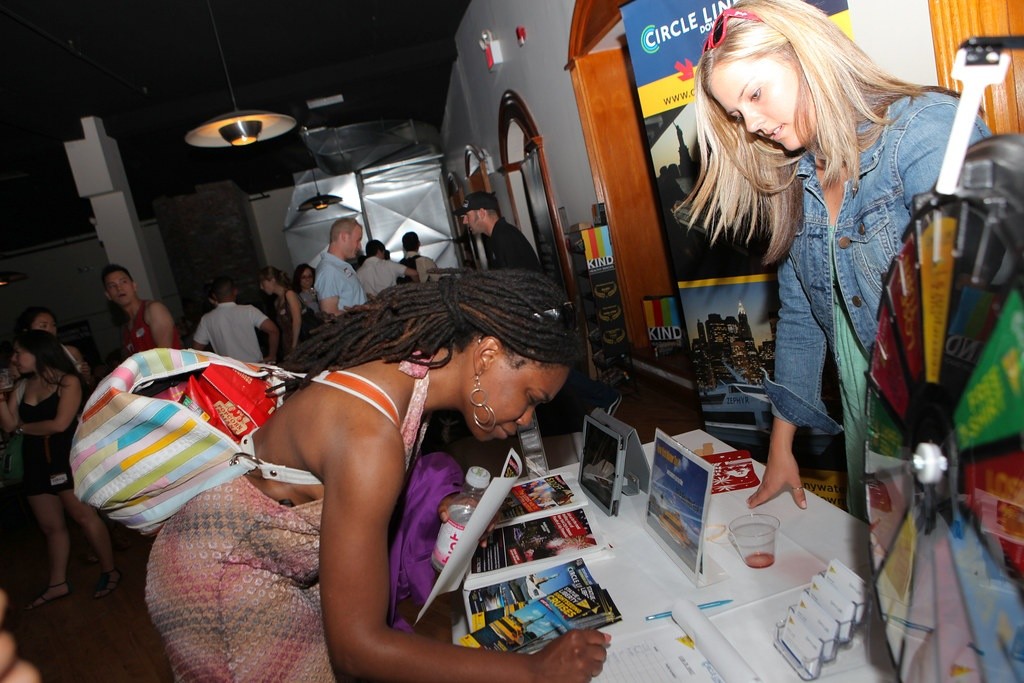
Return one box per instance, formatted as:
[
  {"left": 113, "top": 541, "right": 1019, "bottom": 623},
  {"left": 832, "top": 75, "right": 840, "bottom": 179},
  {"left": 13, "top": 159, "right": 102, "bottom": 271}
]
[{"left": 452, "top": 191, "right": 499, "bottom": 216}]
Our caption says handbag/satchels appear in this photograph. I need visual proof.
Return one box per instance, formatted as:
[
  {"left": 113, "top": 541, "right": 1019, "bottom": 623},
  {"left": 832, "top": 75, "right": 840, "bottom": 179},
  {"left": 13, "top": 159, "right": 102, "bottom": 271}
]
[
  {"left": 0, "top": 431, "right": 25, "bottom": 490},
  {"left": 283, "top": 289, "right": 325, "bottom": 344}
]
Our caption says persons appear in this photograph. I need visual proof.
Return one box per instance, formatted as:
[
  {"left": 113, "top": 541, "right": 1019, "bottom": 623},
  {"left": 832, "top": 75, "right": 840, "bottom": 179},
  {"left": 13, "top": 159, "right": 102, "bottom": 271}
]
[
  {"left": 191, "top": 275, "right": 279, "bottom": 363},
  {"left": 453, "top": 191, "right": 623, "bottom": 415},
  {"left": 684, "top": 1, "right": 995, "bottom": 519},
  {"left": 356, "top": 240, "right": 418, "bottom": 297},
  {"left": 315, "top": 218, "right": 368, "bottom": 317},
  {"left": 103, "top": 264, "right": 181, "bottom": 357},
  {"left": 294, "top": 263, "right": 320, "bottom": 312},
  {"left": 147, "top": 267, "right": 611, "bottom": 683},
  {"left": 0, "top": 307, "right": 134, "bottom": 611},
  {"left": 259, "top": 266, "right": 303, "bottom": 357},
  {"left": 396, "top": 232, "right": 436, "bottom": 284}
]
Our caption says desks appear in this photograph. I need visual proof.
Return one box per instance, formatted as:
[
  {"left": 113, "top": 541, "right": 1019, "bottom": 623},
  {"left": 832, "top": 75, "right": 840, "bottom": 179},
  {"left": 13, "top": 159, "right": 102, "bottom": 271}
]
[{"left": 451, "top": 429, "right": 900, "bottom": 683}]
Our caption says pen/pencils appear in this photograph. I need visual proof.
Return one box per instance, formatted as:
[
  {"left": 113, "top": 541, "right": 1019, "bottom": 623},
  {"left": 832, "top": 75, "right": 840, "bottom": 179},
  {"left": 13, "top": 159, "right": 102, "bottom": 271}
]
[{"left": 645, "top": 600, "right": 737, "bottom": 622}]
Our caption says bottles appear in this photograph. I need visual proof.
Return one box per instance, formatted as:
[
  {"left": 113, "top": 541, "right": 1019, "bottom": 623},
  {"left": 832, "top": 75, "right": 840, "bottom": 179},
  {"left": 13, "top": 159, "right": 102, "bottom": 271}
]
[{"left": 430, "top": 465, "right": 492, "bottom": 579}]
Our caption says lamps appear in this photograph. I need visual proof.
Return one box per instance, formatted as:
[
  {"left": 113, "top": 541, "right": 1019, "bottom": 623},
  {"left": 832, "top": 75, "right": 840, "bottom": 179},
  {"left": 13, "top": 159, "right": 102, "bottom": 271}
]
[
  {"left": 185, "top": 0, "right": 297, "bottom": 148},
  {"left": 479, "top": 29, "right": 503, "bottom": 72},
  {"left": 297, "top": 123, "right": 342, "bottom": 213}
]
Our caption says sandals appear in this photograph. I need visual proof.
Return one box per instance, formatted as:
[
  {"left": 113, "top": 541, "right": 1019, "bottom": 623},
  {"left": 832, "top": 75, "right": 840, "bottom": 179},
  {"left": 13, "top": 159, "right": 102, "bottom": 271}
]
[
  {"left": 91, "top": 566, "right": 124, "bottom": 600},
  {"left": 23, "top": 579, "right": 71, "bottom": 611}
]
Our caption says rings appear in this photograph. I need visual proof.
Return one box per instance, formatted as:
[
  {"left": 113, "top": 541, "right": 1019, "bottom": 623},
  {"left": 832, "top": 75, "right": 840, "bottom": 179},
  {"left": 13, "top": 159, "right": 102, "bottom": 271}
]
[{"left": 792, "top": 487, "right": 802, "bottom": 489}]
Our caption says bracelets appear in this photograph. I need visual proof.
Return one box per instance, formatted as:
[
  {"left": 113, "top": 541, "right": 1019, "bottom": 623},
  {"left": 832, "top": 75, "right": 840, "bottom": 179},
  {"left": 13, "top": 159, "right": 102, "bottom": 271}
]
[
  {"left": 16, "top": 424, "right": 24, "bottom": 434},
  {"left": 0, "top": 398, "right": 7, "bottom": 403}
]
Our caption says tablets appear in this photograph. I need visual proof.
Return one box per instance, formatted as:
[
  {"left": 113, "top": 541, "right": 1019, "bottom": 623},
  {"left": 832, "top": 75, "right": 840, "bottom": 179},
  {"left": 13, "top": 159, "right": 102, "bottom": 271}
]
[{"left": 578, "top": 414, "right": 624, "bottom": 517}]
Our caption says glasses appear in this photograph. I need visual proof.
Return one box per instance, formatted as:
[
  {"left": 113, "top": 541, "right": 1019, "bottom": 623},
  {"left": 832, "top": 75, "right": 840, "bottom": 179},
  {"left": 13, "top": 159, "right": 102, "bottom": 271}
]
[{"left": 701, "top": 8, "right": 763, "bottom": 54}]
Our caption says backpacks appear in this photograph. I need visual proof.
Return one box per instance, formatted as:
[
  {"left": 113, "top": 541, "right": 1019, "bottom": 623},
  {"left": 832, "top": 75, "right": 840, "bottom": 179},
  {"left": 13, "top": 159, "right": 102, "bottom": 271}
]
[
  {"left": 396, "top": 254, "right": 430, "bottom": 285},
  {"left": 69, "top": 348, "right": 401, "bottom": 538}
]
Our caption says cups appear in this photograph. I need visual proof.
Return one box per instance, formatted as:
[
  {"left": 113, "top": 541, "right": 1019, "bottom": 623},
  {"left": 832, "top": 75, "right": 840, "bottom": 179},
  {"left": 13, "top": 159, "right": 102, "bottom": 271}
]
[
  {"left": 0, "top": 368, "right": 14, "bottom": 394},
  {"left": 728, "top": 513, "right": 780, "bottom": 570}
]
